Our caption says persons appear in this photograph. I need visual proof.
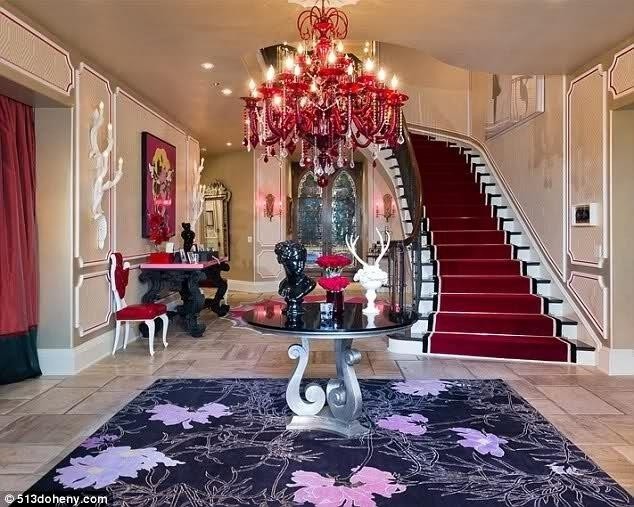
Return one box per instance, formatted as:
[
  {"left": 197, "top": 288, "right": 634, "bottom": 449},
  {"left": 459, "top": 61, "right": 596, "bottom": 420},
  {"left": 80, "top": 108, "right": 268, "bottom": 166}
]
[{"left": 321, "top": 304, "right": 332, "bottom": 317}]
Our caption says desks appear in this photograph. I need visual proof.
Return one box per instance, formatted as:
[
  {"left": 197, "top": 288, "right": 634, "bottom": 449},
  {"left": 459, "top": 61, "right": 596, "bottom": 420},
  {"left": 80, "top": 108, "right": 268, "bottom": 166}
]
[
  {"left": 242, "top": 300, "right": 417, "bottom": 442},
  {"left": 138, "top": 251, "right": 230, "bottom": 337}
]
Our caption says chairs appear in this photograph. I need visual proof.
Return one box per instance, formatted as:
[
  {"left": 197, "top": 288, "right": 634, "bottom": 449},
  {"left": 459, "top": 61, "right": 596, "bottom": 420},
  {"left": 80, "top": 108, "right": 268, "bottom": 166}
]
[{"left": 109, "top": 253, "right": 169, "bottom": 356}]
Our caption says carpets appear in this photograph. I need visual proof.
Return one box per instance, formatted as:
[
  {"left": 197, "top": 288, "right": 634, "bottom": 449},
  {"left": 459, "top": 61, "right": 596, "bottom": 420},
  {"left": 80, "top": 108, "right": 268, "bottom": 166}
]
[{"left": 9, "top": 379, "right": 633, "bottom": 505}]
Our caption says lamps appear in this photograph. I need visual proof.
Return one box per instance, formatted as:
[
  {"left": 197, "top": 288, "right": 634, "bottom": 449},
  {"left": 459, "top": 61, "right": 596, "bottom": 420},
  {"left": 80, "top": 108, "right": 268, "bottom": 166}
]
[{"left": 239, "top": 0, "right": 409, "bottom": 191}]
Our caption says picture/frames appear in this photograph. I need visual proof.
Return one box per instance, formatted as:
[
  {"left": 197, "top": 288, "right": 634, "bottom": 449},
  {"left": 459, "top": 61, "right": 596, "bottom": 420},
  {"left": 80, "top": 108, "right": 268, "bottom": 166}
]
[
  {"left": 141, "top": 131, "right": 176, "bottom": 238},
  {"left": 486, "top": 70, "right": 545, "bottom": 140}
]
